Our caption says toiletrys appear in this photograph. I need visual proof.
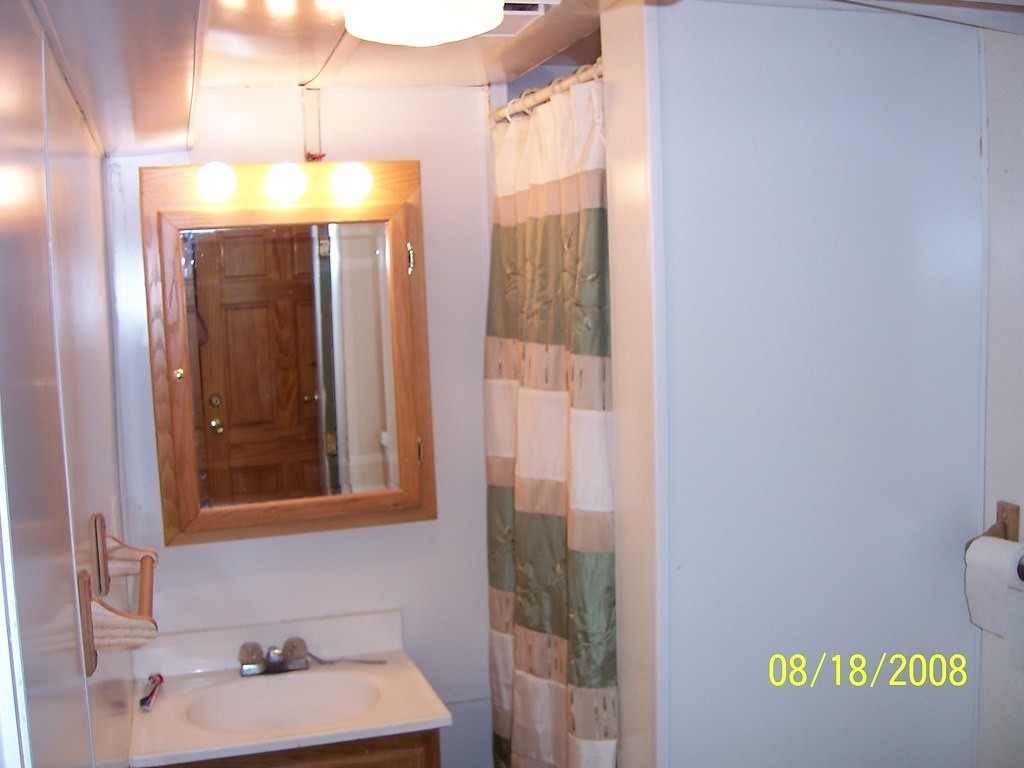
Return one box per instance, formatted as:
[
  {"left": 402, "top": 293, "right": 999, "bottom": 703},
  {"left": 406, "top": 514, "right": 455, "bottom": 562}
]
[{"left": 139, "top": 672, "right": 164, "bottom": 713}]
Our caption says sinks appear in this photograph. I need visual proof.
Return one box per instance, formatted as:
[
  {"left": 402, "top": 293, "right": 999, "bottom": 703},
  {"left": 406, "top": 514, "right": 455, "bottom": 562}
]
[{"left": 182, "top": 665, "right": 385, "bottom": 740}]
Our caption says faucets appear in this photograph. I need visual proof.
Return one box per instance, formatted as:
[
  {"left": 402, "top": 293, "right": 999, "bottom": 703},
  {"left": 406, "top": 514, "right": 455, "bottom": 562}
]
[
  {"left": 238, "top": 640, "right": 266, "bottom": 676},
  {"left": 282, "top": 636, "right": 309, "bottom": 671}
]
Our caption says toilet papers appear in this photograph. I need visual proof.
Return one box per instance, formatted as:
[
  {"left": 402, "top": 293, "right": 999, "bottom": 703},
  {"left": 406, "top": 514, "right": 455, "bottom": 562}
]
[{"left": 965, "top": 535, "right": 1024, "bottom": 638}]
